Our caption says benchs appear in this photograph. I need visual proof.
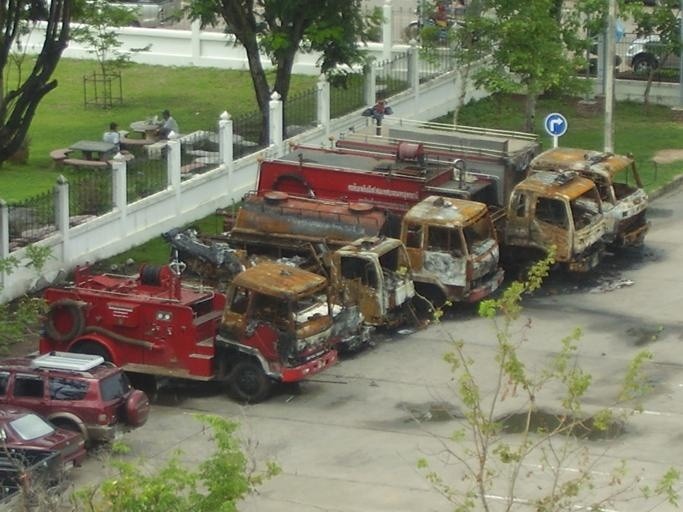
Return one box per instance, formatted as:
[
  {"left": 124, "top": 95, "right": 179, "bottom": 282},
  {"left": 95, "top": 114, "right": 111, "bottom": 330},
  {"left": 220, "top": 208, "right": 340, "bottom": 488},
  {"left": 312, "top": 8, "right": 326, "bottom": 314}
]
[
  {"left": 49, "top": 148, "right": 73, "bottom": 166},
  {"left": 106, "top": 160, "right": 114, "bottom": 165},
  {"left": 63, "top": 158, "right": 108, "bottom": 171},
  {"left": 148, "top": 133, "right": 185, "bottom": 160},
  {"left": 118, "top": 130, "right": 155, "bottom": 145}
]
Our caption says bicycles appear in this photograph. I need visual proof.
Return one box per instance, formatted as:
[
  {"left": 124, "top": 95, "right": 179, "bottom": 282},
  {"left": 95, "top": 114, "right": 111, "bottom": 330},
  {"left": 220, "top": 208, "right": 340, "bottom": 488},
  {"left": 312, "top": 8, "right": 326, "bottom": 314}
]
[{"left": 406, "top": 6, "right": 470, "bottom": 44}]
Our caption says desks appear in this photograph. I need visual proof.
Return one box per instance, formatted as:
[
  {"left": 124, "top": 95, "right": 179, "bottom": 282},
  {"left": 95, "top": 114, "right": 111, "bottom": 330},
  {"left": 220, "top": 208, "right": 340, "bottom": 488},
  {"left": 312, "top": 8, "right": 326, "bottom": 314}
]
[
  {"left": 68, "top": 139, "right": 115, "bottom": 161},
  {"left": 130, "top": 121, "right": 159, "bottom": 139}
]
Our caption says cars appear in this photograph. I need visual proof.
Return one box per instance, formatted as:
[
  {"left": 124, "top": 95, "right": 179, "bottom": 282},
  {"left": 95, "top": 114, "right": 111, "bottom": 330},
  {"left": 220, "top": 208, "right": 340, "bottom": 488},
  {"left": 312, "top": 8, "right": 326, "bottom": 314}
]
[
  {"left": 0, "top": 404, "right": 87, "bottom": 476},
  {"left": 86, "top": 0, "right": 163, "bottom": 28},
  {"left": 625, "top": 36, "right": 683, "bottom": 75}
]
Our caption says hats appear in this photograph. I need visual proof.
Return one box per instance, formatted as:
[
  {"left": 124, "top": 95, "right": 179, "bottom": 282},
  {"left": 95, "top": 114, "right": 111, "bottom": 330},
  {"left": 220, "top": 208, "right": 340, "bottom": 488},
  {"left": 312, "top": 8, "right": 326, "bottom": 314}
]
[
  {"left": 111, "top": 123, "right": 117, "bottom": 129},
  {"left": 161, "top": 110, "right": 171, "bottom": 116}
]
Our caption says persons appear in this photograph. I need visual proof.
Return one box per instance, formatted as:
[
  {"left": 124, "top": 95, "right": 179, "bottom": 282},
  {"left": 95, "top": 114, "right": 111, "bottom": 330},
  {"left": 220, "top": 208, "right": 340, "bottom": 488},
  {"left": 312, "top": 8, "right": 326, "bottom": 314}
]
[
  {"left": 103, "top": 122, "right": 121, "bottom": 151},
  {"left": 154, "top": 110, "right": 179, "bottom": 140}
]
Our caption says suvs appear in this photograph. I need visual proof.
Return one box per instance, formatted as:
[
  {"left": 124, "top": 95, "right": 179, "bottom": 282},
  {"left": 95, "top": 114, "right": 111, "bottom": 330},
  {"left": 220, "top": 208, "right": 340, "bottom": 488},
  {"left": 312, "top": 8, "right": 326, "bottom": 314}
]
[{"left": 0, "top": 351, "right": 150, "bottom": 452}]
